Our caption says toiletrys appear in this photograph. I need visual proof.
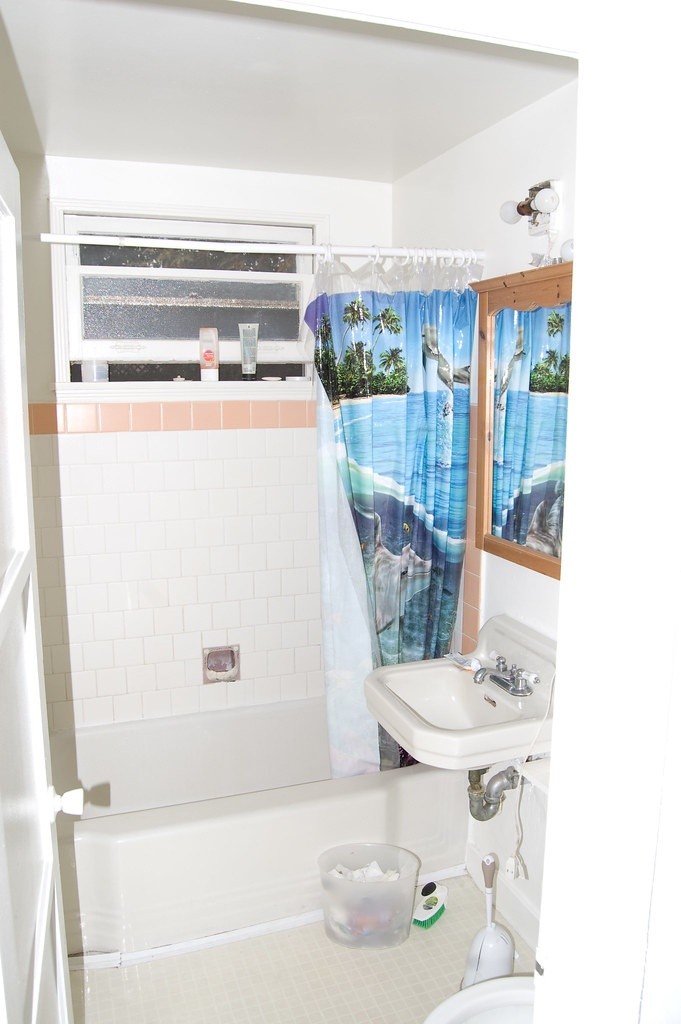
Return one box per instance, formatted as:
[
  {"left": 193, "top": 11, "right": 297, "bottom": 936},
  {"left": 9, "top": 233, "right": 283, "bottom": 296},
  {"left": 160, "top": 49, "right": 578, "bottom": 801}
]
[
  {"left": 80, "top": 359, "right": 110, "bottom": 383},
  {"left": 237, "top": 322, "right": 260, "bottom": 381},
  {"left": 199, "top": 327, "right": 220, "bottom": 381}
]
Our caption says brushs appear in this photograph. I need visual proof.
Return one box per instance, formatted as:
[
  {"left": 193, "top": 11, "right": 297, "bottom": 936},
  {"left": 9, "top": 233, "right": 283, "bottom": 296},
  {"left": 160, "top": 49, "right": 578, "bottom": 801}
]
[{"left": 413, "top": 881, "right": 448, "bottom": 929}]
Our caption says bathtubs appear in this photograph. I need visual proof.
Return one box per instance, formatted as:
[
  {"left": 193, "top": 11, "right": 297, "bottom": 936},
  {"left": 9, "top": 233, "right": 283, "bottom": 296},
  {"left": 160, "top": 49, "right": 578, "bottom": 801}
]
[{"left": 49, "top": 692, "right": 470, "bottom": 954}]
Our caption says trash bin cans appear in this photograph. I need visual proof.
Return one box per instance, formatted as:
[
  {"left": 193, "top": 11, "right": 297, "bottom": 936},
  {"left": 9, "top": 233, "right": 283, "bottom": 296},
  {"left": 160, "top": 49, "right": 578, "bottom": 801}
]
[{"left": 319, "top": 842, "right": 423, "bottom": 950}]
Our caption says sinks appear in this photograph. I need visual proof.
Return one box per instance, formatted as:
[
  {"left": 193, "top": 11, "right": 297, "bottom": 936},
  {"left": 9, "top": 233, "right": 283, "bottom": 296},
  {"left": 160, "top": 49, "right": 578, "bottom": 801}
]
[{"left": 363, "top": 614, "right": 558, "bottom": 771}]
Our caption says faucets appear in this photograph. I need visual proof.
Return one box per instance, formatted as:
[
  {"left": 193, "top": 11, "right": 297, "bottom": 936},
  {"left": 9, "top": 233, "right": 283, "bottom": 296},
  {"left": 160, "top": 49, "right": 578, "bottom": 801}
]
[{"left": 472, "top": 664, "right": 517, "bottom": 685}]
